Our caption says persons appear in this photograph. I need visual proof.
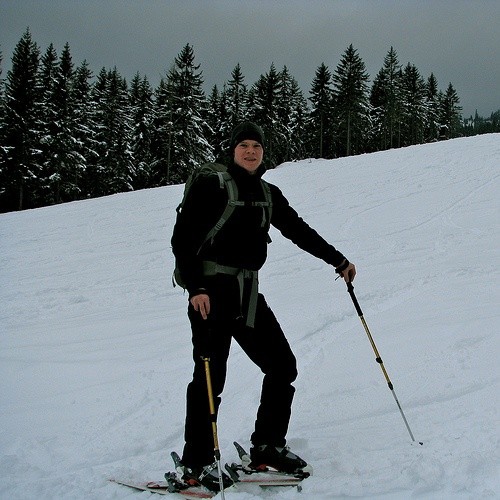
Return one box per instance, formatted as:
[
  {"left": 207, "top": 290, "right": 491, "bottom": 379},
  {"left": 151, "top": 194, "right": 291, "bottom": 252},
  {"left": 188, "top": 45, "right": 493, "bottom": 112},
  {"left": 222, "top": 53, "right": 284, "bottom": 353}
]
[{"left": 171, "top": 121, "right": 357, "bottom": 492}]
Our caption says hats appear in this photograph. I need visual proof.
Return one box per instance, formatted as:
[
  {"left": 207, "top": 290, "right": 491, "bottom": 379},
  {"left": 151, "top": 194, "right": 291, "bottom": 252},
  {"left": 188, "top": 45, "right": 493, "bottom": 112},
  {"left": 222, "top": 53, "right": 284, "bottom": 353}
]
[{"left": 230, "top": 121, "right": 267, "bottom": 152}]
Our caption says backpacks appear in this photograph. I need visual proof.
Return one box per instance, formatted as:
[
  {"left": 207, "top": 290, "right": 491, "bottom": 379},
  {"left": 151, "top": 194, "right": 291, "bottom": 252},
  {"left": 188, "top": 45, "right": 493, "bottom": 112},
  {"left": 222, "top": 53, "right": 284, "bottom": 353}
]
[{"left": 171, "top": 162, "right": 273, "bottom": 328}]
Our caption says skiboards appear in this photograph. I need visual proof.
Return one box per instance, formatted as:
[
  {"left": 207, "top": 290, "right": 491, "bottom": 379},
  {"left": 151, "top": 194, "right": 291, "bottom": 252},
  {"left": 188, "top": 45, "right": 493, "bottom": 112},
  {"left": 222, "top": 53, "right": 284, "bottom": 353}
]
[
  {"left": 107, "top": 478, "right": 217, "bottom": 500},
  {"left": 238, "top": 465, "right": 303, "bottom": 485}
]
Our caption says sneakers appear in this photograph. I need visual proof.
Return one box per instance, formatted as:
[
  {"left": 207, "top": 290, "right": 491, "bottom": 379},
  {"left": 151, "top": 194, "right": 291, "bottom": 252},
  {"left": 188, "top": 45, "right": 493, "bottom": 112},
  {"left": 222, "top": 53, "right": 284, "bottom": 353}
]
[
  {"left": 179, "top": 460, "right": 240, "bottom": 495},
  {"left": 250, "top": 444, "right": 314, "bottom": 478}
]
